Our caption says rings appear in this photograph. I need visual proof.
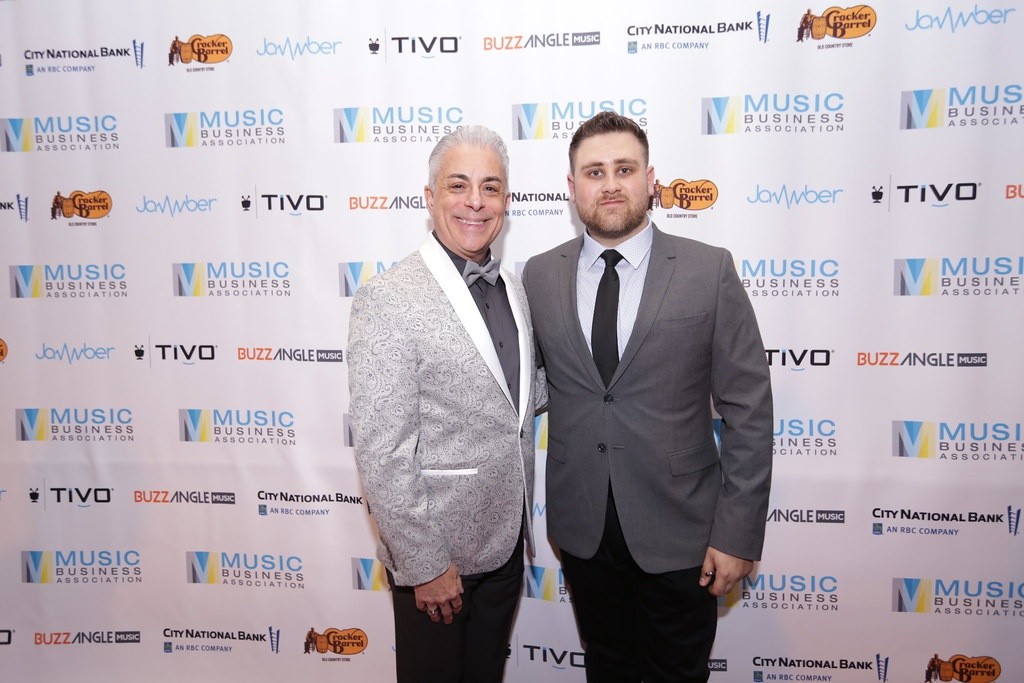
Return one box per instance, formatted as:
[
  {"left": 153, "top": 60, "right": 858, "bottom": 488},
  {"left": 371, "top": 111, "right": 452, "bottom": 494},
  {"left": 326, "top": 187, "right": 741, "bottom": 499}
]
[{"left": 428, "top": 608, "right": 438, "bottom": 614}]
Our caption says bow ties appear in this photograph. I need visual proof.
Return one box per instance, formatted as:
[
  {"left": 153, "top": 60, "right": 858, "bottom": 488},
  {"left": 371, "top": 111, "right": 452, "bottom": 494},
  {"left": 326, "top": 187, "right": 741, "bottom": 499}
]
[{"left": 462, "top": 259, "right": 501, "bottom": 288}]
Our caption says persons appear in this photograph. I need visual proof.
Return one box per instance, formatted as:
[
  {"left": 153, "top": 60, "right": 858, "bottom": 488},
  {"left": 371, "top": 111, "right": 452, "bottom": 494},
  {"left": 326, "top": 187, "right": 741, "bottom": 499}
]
[
  {"left": 347, "top": 126, "right": 549, "bottom": 683},
  {"left": 518, "top": 110, "right": 775, "bottom": 683}
]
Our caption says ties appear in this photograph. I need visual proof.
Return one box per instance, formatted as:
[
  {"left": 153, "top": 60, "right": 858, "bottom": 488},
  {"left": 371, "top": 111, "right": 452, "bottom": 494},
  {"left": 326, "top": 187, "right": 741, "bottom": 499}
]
[{"left": 590, "top": 250, "right": 624, "bottom": 389}]
